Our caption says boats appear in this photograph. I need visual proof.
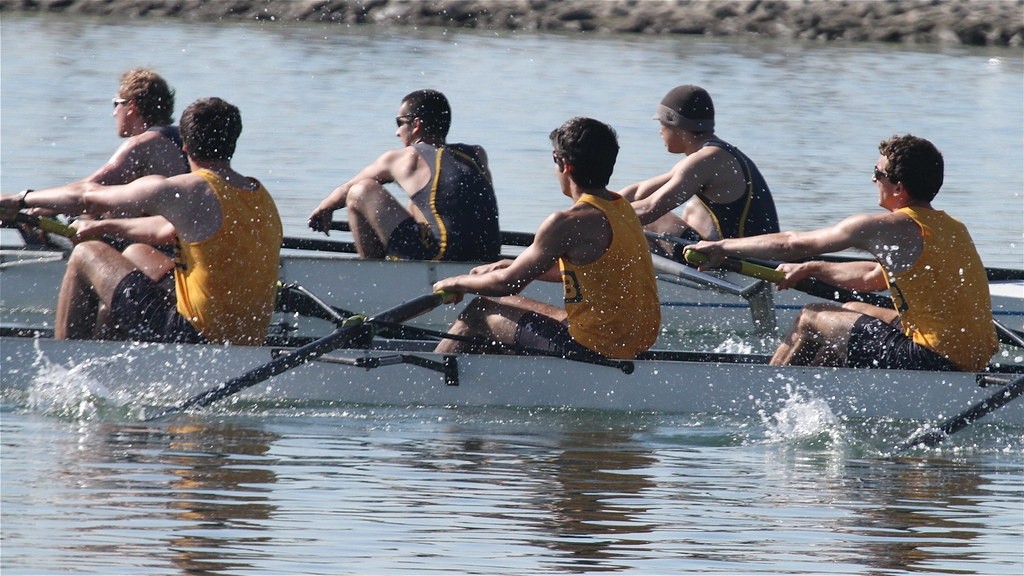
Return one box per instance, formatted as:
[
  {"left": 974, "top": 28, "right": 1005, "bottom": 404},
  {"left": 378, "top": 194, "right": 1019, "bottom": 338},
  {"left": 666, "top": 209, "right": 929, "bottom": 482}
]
[
  {"left": 0, "top": 244, "right": 1024, "bottom": 358},
  {"left": 0, "top": 328, "right": 1024, "bottom": 436}
]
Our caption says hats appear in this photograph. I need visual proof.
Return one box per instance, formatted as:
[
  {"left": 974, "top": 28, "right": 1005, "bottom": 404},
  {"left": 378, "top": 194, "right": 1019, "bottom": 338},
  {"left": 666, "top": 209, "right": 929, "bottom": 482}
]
[{"left": 652, "top": 85, "right": 715, "bottom": 131}]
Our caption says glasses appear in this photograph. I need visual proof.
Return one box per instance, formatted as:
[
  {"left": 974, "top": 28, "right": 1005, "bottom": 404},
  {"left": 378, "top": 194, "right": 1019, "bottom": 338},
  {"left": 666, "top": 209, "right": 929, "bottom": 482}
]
[
  {"left": 113, "top": 98, "right": 129, "bottom": 108},
  {"left": 874, "top": 166, "right": 889, "bottom": 180},
  {"left": 396, "top": 115, "right": 423, "bottom": 127},
  {"left": 553, "top": 150, "right": 568, "bottom": 163}
]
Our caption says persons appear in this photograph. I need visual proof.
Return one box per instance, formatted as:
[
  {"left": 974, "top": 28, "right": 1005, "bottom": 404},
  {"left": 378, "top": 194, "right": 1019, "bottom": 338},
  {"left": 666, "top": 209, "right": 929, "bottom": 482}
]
[
  {"left": 683, "top": 134, "right": 999, "bottom": 372},
  {"left": 307, "top": 89, "right": 502, "bottom": 261},
  {"left": 1, "top": 98, "right": 284, "bottom": 346},
  {"left": 617, "top": 85, "right": 780, "bottom": 268},
  {"left": 24, "top": 69, "right": 191, "bottom": 254},
  {"left": 434, "top": 117, "right": 661, "bottom": 359}
]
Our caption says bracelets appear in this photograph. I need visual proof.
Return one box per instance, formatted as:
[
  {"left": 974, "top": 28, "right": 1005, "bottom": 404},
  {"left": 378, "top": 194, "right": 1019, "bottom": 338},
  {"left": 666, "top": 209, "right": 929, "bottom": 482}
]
[{"left": 19, "top": 189, "right": 33, "bottom": 209}]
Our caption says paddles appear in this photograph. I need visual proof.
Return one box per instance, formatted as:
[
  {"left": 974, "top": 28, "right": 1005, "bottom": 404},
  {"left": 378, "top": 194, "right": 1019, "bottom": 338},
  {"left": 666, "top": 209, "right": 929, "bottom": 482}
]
[
  {"left": 683, "top": 247, "right": 1024, "bottom": 350},
  {"left": 168, "top": 289, "right": 467, "bottom": 409},
  {"left": 307, "top": 219, "right": 535, "bottom": 248},
  {"left": 893, "top": 374, "right": 1024, "bottom": 454},
  {"left": 0, "top": 206, "right": 180, "bottom": 260}
]
